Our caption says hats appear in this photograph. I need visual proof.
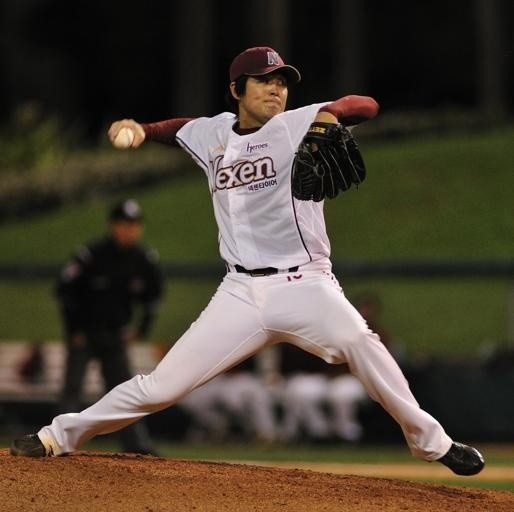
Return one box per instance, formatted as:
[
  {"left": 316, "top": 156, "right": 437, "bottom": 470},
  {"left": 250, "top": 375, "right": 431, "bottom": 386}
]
[
  {"left": 228, "top": 47, "right": 303, "bottom": 84},
  {"left": 107, "top": 196, "right": 149, "bottom": 228}
]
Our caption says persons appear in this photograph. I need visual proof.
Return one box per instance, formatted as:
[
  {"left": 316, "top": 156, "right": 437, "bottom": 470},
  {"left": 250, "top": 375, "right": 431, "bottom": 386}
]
[
  {"left": 55, "top": 198, "right": 165, "bottom": 459},
  {"left": 170, "top": 294, "right": 387, "bottom": 446},
  {"left": 10, "top": 47, "right": 483, "bottom": 475}
]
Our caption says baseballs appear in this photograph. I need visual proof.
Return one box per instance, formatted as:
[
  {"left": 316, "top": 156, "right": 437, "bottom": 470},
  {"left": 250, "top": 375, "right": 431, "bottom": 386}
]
[{"left": 112, "top": 128, "right": 136, "bottom": 149}]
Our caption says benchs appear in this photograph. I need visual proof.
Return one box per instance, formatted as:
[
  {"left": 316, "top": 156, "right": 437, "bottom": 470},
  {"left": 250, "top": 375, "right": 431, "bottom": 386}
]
[{"left": 0, "top": 337, "right": 289, "bottom": 443}]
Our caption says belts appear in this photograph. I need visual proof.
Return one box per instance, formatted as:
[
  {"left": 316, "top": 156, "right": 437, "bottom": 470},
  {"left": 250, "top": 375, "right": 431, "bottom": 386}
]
[{"left": 230, "top": 261, "right": 301, "bottom": 276}]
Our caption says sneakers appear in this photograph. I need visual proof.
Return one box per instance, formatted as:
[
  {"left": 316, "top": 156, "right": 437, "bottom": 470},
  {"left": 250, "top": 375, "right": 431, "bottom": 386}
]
[
  {"left": 437, "top": 440, "right": 486, "bottom": 475},
  {"left": 10, "top": 432, "right": 67, "bottom": 457}
]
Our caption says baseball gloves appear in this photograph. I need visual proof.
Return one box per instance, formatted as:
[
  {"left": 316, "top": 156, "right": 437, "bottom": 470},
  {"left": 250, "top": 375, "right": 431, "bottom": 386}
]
[{"left": 291, "top": 120, "right": 367, "bottom": 201}]
